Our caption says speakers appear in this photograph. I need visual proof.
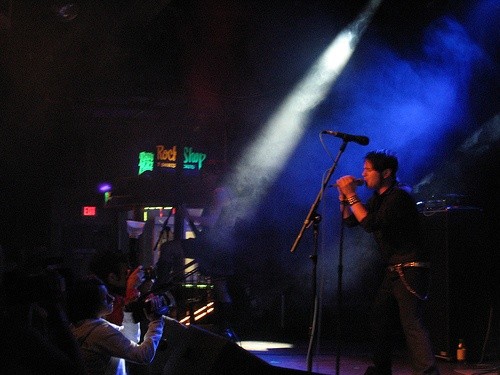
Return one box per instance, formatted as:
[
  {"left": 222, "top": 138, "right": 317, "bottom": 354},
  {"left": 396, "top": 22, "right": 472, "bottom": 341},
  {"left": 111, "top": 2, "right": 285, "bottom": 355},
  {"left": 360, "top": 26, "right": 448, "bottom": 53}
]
[{"left": 142, "top": 315, "right": 276, "bottom": 375}]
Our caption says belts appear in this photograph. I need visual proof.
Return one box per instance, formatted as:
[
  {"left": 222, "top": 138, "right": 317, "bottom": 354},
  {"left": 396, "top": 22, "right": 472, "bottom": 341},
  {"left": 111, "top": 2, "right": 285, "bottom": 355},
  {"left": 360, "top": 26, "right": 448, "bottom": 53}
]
[{"left": 386, "top": 261, "right": 428, "bottom": 301}]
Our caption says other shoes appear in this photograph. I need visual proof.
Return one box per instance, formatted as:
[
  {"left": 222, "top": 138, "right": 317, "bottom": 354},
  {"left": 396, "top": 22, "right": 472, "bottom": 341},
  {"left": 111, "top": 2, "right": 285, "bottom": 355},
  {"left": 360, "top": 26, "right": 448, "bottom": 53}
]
[
  {"left": 423, "top": 366, "right": 440, "bottom": 375},
  {"left": 363, "top": 366, "right": 392, "bottom": 375}
]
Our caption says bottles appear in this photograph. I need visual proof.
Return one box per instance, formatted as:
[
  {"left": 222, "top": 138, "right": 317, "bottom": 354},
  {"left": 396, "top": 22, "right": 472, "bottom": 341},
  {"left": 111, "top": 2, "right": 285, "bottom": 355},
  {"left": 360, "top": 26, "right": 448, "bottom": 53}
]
[{"left": 455, "top": 338, "right": 466, "bottom": 371}]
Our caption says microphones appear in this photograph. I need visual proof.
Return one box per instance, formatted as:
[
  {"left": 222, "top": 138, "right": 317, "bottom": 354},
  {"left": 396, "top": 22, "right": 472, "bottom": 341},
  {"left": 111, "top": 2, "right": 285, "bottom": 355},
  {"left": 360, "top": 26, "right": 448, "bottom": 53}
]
[
  {"left": 329, "top": 179, "right": 363, "bottom": 187},
  {"left": 322, "top": 131, "right": 369, "bottom": 146}
]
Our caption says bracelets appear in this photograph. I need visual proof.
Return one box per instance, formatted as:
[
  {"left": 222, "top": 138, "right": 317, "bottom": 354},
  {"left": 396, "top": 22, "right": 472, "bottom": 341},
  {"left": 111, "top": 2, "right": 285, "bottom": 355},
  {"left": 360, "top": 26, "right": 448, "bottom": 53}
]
[
  {"left": 340, "top": 200, "right": 347, "bottom": 204},
  {"left": 345, "top": 194, "right": 360, "bottom": 205}
]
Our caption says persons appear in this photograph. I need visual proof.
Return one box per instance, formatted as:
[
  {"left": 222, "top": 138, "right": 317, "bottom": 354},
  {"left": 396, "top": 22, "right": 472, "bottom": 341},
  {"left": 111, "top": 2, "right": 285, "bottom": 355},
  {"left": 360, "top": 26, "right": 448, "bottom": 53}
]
[
  {"left": 68, "top": 266, "right": 166, "bottom": 375},
  {"left": 336, "top": 153, "right": 440, "bottom": 375}
]
